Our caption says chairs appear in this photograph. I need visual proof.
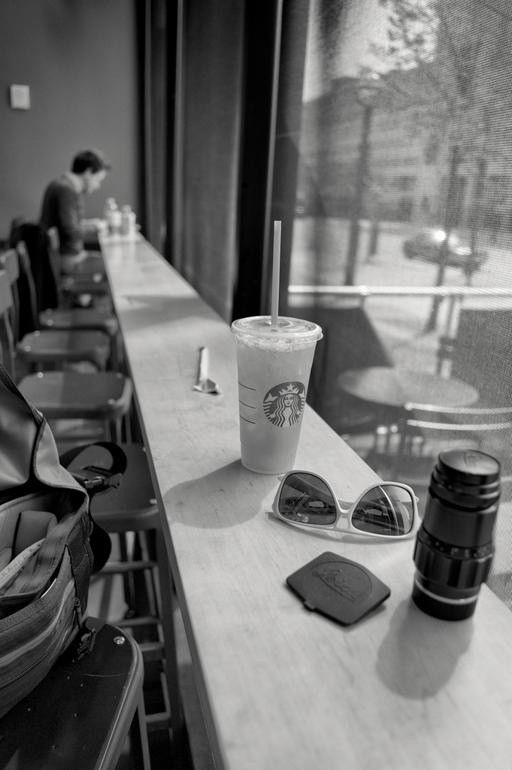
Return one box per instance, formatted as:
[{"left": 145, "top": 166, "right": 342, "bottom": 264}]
[{"left": 0, "top": 212, "right": 511, "bottom": 769}]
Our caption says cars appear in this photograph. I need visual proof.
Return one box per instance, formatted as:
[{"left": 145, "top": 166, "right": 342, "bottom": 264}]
[{"left": 398, "top": 227, "right": 491, "bottom": 272}]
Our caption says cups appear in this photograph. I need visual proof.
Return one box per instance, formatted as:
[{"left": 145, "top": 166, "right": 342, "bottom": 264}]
[{"left": 231, "top": 315, "right": 325, "bottom": 477}]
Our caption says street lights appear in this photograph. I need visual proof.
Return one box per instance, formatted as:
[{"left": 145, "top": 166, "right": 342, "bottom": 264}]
[{"left": 343, "top": 63, "right": 383, "bottom": 287}]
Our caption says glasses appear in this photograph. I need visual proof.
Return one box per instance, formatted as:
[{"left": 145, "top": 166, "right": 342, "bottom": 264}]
[{"left": 272, "top": 470, "right": 418, "bottom": 539}]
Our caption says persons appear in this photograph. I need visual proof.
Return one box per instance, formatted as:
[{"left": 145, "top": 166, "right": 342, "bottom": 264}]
[{"left": 38, "top": 148, "right": 111, "bottom": 276}]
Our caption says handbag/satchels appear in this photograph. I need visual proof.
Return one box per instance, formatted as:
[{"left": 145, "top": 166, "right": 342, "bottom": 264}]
[{"left": 0, "top": 362, "right": 128, "bottom": 719}]
